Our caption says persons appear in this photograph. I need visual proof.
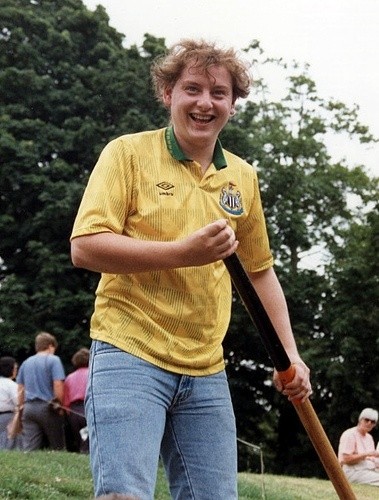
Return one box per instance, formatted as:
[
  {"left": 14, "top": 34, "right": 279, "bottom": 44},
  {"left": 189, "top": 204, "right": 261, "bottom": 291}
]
[
  {"left": 337, "top": 408, "right": 379, "bottom": 487},
  {"left": 70, "top": 36, "right": 314, "bottom": 500},
  {"left": 17, "top": 332, "right": 68, "bottom": 451},
  {"left": 58, "top": 347, "right": 92, "bottom": 454},
  {"left": 0, "top": 356, "right": 24, "bottom": 451}
]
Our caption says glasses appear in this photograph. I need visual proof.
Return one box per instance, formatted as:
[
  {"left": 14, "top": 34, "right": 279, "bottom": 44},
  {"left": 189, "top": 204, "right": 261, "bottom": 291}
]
[{"left": 364, "top": 418, "right": 375, "bottom": 424}]
